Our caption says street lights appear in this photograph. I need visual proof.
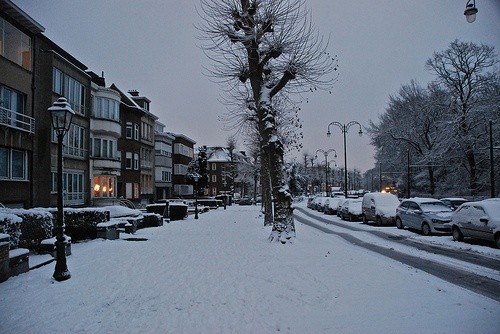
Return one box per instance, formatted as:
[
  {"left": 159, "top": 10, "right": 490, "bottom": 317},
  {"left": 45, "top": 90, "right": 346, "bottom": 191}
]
[
  {"left": 489, "top": 119, "right": 495, "bottom": 198},
  {"left": 327, "top": 121, "right": 363, "bottom": 199},
  {"left": 48, "top": 96, "right": 71, "bottom": 282},
  {"left": 314, "top": 148, "right": 338, "bottom": 197},
  {"left": 193, "top": 173, "right": 201, "bottom": 219},
  {"left": 407, "top": 149, "right": 410, "bottom": 199}
]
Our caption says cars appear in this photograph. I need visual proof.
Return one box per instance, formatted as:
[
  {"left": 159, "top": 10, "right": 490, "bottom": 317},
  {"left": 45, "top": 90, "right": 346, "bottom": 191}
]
[
  {"left": 362, "top": 192, "right": 401, "bottom": 226},
  {"left": 396, "top": 198, "right": 455, "bottom": 236},
  {"left": 451, "top": 200, "right": 500, "bottom": 246},
  {"left": 338, "top": 199, "right": 365, "bottom": 223},
  {"left": 323, "top": 198, "right": 339, "bottom": 215},
  {"left": 441, "top": 197, "right": 470, "bottom": 212},
  {"left": 231, "top": 193, "right": 262, "bottom": 206},
  {"left": 307, "top": 186, "right": 345, "bottom": 212}
]
